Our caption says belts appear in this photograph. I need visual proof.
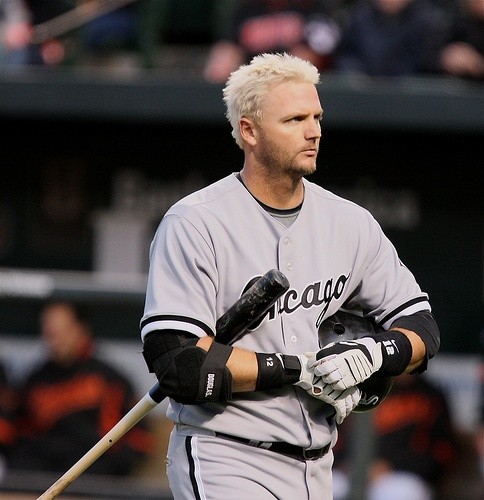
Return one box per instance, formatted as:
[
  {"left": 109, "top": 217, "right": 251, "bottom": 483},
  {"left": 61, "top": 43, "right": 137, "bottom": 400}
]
[{"left": 215, "top": 430, "right": 330, "bottom": 459}]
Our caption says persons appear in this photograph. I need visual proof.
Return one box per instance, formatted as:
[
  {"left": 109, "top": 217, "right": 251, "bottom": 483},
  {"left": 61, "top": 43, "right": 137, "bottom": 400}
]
[
  {"left": 368, "top": 376, "right": 483, "bottom": 500},
  {"left": 12, "top": 288, "right": 157, "bottom": 478},
  {"left": 0, "top": 0, "right": 484, "bottom": 87},
  {"left": 139, "top": 51, "right": 441, "bottom": 500}
]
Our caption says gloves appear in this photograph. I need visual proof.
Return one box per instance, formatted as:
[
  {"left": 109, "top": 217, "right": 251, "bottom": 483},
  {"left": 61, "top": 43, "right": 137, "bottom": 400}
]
[
  {"left": 294, "top": 356, "right": 361, "bottom": 423},
  {"left": 304, "top": 339, "right": 383, "bottom": 391}
]
